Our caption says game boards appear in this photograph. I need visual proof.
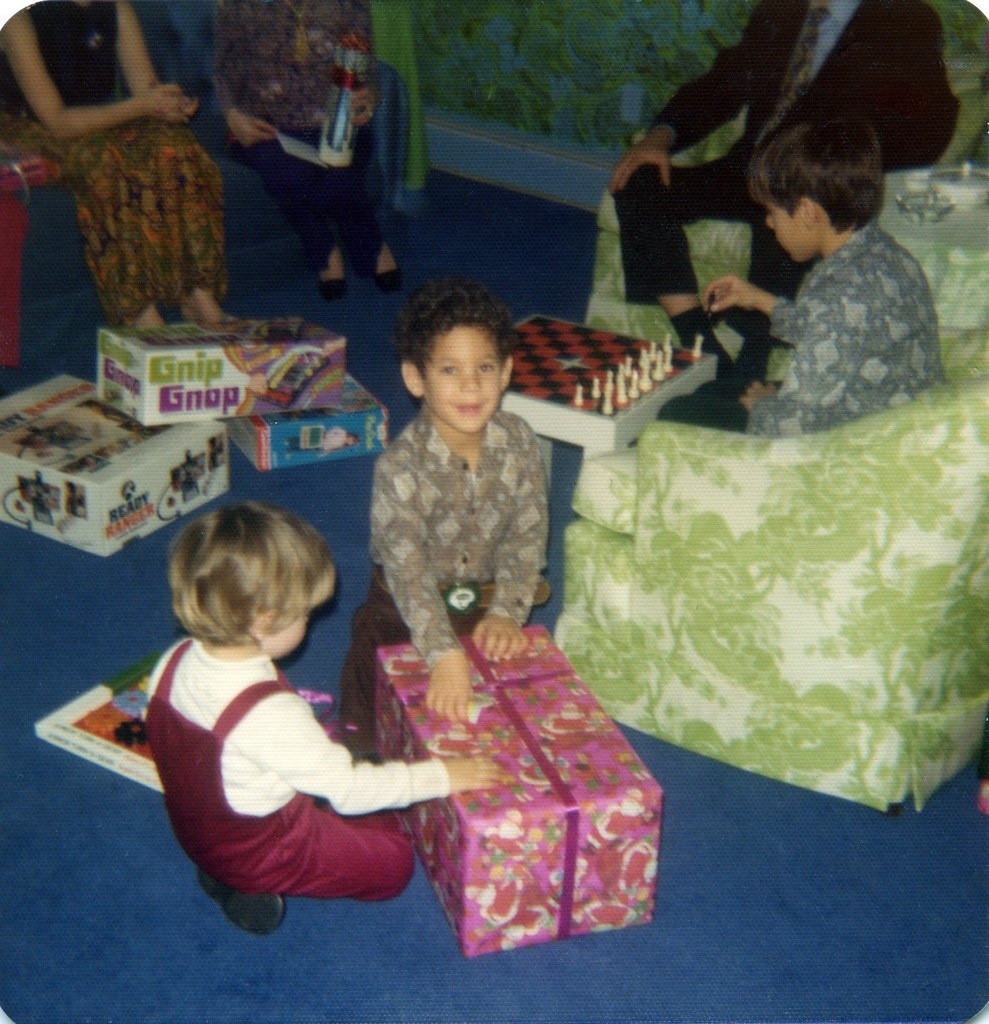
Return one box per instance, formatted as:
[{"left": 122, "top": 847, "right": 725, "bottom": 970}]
[{"left": 500, "top": 310, "right": 719, "bottom": 451}]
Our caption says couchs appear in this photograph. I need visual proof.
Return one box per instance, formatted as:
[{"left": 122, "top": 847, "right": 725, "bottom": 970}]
[
  {"left": 556, "top": 328, "right": 989, "bottom": 818},
  {"left": 584, "top": 0, "right": 989, "bottom": 382},
  {"left": 0, "top": 0, "right": 413, "bottom": 337}
]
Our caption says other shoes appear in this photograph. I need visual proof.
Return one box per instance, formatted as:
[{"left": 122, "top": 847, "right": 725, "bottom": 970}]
[
  {"left": 375, "top": 267, "right": 401, "bottom": 288},
  {"left": 318, "top": 276, "right": 346, "bottom": 298},
  {"left": 196, "top": 867, "right": 283, "bottom": 935}
]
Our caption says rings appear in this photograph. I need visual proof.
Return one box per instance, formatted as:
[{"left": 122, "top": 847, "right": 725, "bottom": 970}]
[{"left": 368, "top": 111, "right": 375, "bottom": 117}]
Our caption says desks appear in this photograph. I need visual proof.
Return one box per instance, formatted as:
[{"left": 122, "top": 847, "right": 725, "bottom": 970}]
[{"left": 880, "top": 160, "right": 989, "bottom": 329}]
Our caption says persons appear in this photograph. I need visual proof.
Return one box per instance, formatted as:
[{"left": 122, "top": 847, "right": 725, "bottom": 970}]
[
  {"left": 655, "top": 101, "right": 943, "bottom": 441},
  {"left": 333, "top": 291, "right": 553, "bottom": 760},
  {"left": 146, "top": 502, "right": 502, "bottom": 936},
  {"left": 608, "top": 0, "right": 961, "bottom": 401},
  {"left": 212, "top": 0, "right": 401, "bottom": 298},
  {"left": 1, "top": 0, "right": 244, "bottom": 334}
]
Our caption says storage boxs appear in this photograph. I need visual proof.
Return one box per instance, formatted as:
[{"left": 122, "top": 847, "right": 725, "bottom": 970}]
[
  {"left": 369, "top": 621, "right": 664, "bottom": 958},
  {"left": 223, "top": 371, "right": 389, "bottom": 472},
  {"left": 97, "top": 316, "right": 348, "bottom": 427},
  {"left": 0, "top": 373, "right": 231, "bottom": 558}
]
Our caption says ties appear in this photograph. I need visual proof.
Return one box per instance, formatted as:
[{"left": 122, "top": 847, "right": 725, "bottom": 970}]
[
  {"left": 285, "top": 0, "right": 311, "bottom": 65},
  {"left": 755, "top": 7, "right": 828, "bottom": 145}
]
